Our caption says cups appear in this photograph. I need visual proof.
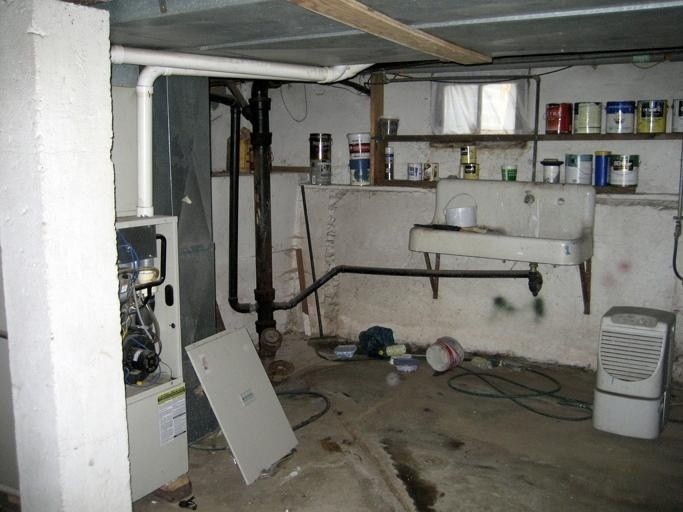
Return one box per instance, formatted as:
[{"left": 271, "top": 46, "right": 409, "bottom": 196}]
[{"left": 596, "top": 151, "right": 611, "bottom": 187}]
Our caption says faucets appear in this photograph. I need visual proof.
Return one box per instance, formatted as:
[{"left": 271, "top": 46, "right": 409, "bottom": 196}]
[{"left": 524, "top": 190, "right": 533, "bottom": 203}]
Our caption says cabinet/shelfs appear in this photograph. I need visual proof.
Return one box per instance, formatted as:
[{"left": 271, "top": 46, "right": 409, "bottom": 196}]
[{"left": 368, "top": 64, "right": 682, "bottom": 199}]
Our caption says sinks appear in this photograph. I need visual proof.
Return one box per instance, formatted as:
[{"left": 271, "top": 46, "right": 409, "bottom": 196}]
[{"left": 408, "top": 225, "right": 594, "bottom": 266}]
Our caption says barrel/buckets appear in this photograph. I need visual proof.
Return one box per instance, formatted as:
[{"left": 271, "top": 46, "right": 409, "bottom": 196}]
[
  {"left": 426, "top": 337, "right": 465, "bottom": 372},
  {"left": 443, "top": 193, "right": 477, "bottom": 228},
  {"left": 348, "top": 159, "right": 370, "bottom": 185},
  {"left": 346, "top": 133, "right": 370, "bottom": 159},
  {"left": 545, "top": 99, "right": 683, "bottom": 134},
  {"left": 565, "top": 154, "right": 592, "bottom": 184},
  {"left": 609, "top": 155, "right": 638, "bottom": 187},
  {"left": 309, "top": 133, "right": 331, "bottom": 184}
]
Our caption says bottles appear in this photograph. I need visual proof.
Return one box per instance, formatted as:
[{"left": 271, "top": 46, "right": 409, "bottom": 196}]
[
  {"left": 470, "top": 356, "right": 493, "bottom": 371},
  {"left": 378, "top": 343, "right": 410, "bottom": 357}
]
